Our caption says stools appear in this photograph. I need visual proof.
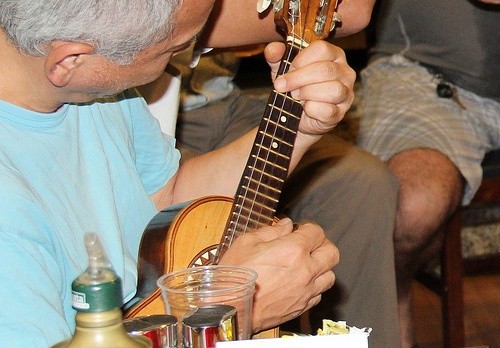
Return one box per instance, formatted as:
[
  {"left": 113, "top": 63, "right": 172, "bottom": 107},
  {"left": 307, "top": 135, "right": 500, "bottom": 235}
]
[{"left": 401, "top": 171, "right": 500, "bottom": 348}]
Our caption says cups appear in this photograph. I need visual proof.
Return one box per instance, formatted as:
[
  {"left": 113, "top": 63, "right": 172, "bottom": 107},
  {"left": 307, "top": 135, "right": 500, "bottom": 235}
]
[{"left": 156, "top": 265, "right": 259, "bottom": 348}]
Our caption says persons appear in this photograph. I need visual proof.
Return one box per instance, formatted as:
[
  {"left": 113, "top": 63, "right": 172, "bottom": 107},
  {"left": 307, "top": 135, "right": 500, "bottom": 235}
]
[
  {"left": 342, "top": 0, "right": 500, "bottom": 348},
  {"left": 146, "top": 0, "right": 402, "bottom": 348},
  {"left": 0, "top": 0, "right": 356, "bottom": 348}
]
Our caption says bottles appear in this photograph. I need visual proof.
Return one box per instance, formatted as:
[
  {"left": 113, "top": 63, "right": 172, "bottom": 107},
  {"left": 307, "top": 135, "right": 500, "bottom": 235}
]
[{"left": 52, "top": 231, "right": 153, "bottom": 348}]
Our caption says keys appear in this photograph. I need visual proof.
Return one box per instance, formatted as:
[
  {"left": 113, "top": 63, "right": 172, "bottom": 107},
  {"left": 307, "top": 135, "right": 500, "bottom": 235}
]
[{"left": 430, "top": 72, "right": 467, "bottom": 111}]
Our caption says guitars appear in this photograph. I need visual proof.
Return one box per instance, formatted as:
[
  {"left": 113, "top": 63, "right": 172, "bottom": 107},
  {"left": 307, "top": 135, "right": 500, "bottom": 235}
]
[{"left": 121, "top": 0, "right": 342, "bottom": 340}]
